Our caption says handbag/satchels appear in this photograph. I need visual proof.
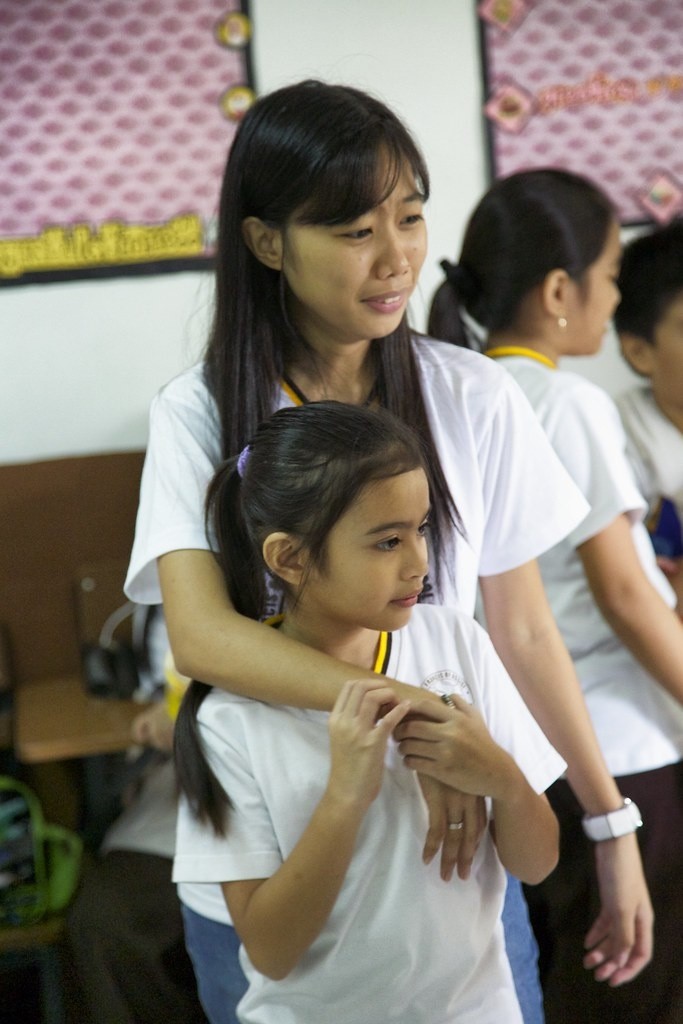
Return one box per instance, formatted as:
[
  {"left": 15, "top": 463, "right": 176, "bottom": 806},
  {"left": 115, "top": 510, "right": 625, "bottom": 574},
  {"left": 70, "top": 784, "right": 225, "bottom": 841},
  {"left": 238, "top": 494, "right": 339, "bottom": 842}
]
[{"left": 79, "top": 596, "right": 151, "bottom": 702}]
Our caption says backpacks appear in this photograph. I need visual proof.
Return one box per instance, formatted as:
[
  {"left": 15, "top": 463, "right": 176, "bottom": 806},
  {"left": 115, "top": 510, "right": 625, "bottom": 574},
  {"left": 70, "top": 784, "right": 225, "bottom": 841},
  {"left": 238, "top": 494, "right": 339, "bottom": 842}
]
[{"left": 0, "top": 777, "right": 83, "bottom": 934}]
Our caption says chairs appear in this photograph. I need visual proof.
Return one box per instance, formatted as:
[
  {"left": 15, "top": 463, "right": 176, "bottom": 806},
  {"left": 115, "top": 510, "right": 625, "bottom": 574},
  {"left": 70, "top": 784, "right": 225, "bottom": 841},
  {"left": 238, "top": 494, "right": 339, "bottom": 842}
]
[{"left": 2, "top": 921, "right": 85, "bottom": 1024}]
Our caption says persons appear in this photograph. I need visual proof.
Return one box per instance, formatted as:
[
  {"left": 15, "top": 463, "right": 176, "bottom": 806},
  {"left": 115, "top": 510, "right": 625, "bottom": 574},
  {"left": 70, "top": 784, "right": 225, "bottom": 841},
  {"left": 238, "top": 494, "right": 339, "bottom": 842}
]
[
  {"left": 72, "top": 609, "right": 199, "bottom": 1024},
  {"left": 123, "top": 79, "right": 682, "bottom": 1024},
  {"left": 170, "top": 401, "right": 568, "bottom": 1024}
]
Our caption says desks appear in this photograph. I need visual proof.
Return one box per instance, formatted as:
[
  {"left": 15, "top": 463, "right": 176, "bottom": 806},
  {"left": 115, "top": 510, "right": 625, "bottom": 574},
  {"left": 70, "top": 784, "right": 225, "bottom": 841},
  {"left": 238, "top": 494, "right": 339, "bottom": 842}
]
[{"left": 9, "top": 681, "right": 163, "bottom": 850}]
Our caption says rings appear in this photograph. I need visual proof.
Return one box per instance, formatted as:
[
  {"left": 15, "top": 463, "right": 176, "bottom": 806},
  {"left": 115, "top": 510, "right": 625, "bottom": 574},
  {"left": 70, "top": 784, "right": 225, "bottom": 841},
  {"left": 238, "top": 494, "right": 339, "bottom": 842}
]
[{"left": 446, "top": 820, "right": 464, "bottom": 830}]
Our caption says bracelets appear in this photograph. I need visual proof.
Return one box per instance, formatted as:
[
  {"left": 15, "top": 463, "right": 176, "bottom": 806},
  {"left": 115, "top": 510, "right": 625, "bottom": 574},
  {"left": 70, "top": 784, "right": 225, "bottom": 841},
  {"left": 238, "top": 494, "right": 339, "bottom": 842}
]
[
  {"left": 583, "top": 795, "right": 643, "bottom": 841},
  {"left": 441, "top": 694, "right": 456, "bottom": 710}
]
